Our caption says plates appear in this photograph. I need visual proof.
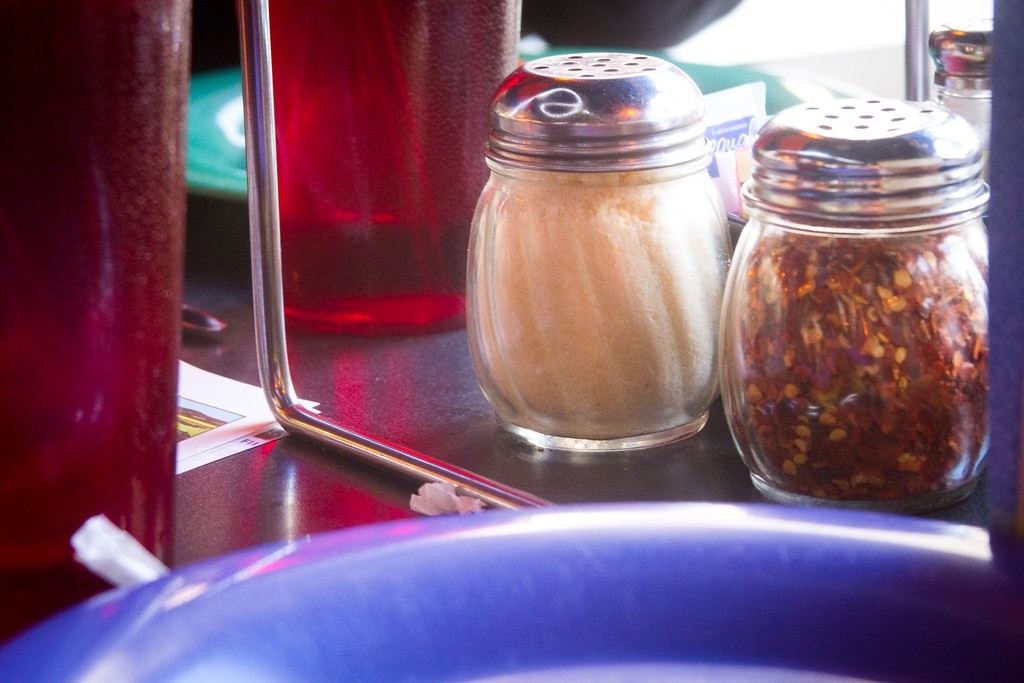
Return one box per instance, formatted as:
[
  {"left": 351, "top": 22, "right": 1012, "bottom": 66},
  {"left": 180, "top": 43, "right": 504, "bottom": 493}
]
[{"left": 188, "top": 46, "right": 804, "bottom": 201}]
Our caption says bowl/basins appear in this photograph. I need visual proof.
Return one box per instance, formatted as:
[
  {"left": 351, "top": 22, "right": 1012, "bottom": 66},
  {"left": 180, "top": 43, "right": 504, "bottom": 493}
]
[{"left": 1, "top": 502, "right": 1024, "bottom": 683}]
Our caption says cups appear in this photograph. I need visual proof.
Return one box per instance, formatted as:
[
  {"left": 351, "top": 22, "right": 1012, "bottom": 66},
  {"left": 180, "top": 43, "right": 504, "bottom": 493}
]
[
  {"left": 0, "top": 1, "right": 194, "bottom": 648},
  {"left": 269, "top": 0, "right": 523, "bottom": 335}
]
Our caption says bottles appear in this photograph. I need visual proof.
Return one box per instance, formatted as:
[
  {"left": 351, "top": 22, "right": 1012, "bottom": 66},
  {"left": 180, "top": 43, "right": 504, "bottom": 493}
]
[
  {"left": 928, "top": 19, "right": 994, "bottom": 227},
  {"left": 717, "top": 96, "right": 990, "bottom": 510},
  {"left": 467, "top": 52, "right": 734, "bottom": 453}
]
